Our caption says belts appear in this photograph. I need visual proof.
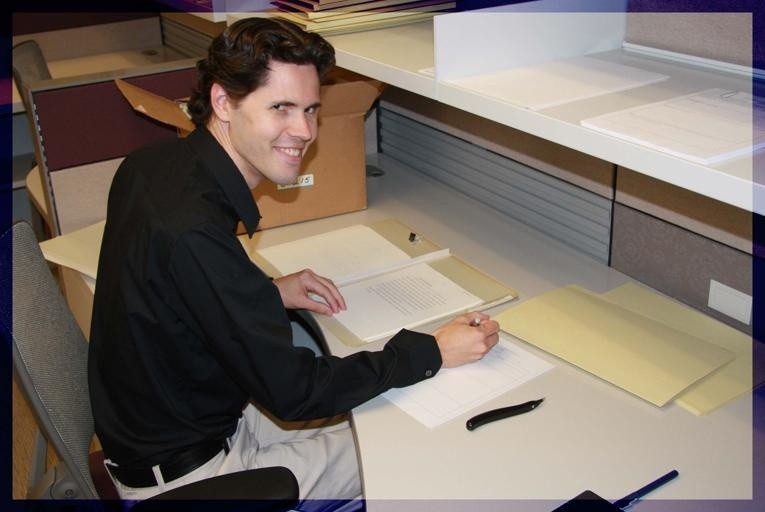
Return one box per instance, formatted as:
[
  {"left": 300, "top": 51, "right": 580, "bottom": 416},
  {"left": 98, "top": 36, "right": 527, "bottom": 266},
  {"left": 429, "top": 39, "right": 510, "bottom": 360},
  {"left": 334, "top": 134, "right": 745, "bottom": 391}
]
[{"left": 105, "top": 438, "right": 224, "bottom": 488}]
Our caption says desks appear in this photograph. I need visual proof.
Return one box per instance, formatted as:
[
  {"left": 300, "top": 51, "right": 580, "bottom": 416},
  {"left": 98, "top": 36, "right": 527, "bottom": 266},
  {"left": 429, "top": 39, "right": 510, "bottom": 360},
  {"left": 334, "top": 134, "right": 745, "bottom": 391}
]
[
  {"left": 12, "top": 45, "right": 192, "bottom": 114},
  {"left": 51, "top": 153, "right": 765, "bottom": 511}
]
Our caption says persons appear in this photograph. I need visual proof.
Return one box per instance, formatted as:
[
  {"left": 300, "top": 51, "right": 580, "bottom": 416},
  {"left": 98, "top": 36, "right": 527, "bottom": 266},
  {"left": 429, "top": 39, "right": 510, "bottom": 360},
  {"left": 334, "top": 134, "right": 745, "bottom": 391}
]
[
  {"left": 86, "top": 17, "right": 499, "bottom": 500},
  {"left": 264, "top": 1, "right": 456, "bottom": 36}
]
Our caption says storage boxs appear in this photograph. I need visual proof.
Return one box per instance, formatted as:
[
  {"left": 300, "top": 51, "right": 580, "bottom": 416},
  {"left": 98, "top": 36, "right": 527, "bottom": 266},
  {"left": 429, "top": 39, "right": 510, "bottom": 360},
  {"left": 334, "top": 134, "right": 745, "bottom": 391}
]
[{"left": 115, "top": 77, "right": 384, "bottom": 235}]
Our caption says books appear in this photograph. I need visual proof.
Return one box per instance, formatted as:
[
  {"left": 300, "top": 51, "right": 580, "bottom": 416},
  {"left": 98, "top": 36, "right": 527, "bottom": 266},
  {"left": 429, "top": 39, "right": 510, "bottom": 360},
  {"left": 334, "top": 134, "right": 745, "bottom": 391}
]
[
  {"left": 258, "top": 224, "right": 485, "bottom": 344},
  {"left": 39, "top": 219, "right": 107, "bottom": 279},
  {"left": 580, "top": 86, "right": 765, "bottom": 166},
  {"left": 449, "top": 55, "right": 668, "bottom": 113}
]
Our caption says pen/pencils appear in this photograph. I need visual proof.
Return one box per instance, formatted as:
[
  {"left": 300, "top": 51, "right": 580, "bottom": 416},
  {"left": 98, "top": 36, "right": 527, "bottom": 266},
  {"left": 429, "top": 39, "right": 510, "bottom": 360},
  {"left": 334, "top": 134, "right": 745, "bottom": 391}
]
[
  {"left": 613, "top": 469, "right": 679, "bottom": 510},
  {"left": 473, "top": 318, "right": 481, "bottom": 327}
]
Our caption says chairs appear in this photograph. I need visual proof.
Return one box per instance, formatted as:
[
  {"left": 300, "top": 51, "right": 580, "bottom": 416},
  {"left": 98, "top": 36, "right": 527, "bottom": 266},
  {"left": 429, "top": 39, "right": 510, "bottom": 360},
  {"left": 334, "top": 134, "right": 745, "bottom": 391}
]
[
  {"left": 12, "top": 222, "right": 300, "bottom": 511},
  {"left": 12, "top": 40, "right": 54, "bottom": 119}
]
